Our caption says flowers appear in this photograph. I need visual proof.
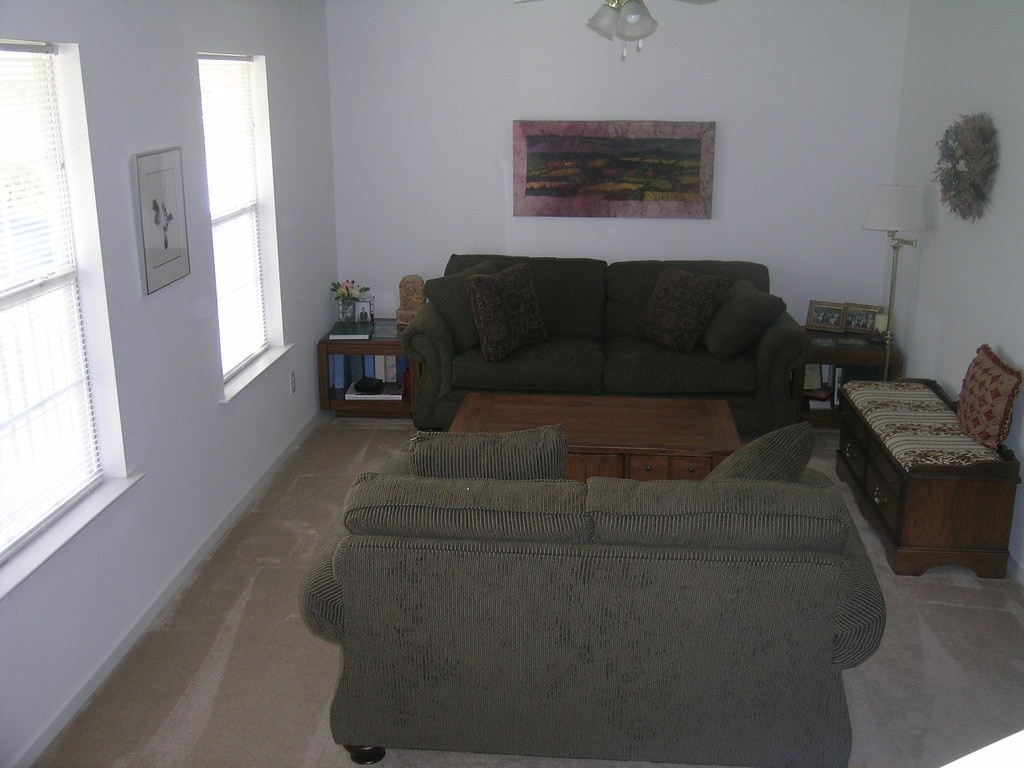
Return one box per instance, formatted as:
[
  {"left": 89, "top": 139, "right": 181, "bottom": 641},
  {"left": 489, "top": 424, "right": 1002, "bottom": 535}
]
[
  {"left": 930, "top": 113, "right": 999, "bottom": 225},
  {"left": 331, "top": 277, "right": 372, "bottom": 299}
]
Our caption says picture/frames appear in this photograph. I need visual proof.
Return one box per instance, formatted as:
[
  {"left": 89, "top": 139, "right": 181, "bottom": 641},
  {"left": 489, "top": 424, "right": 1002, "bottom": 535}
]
[
  {"left": 355, "top": 301, "right": 372, "bottom": 323},
  {"left": 511, "top": 119, "right": 717, "bottom": 221},
  {"left": 844, "top": 303, "right": 884, "bottom": 336},
  {"left": 806, "top": 299, "right": 848, "bottom": 334},
  {"left": 131, "top": 145, "right": 192, "bottom": 300}
]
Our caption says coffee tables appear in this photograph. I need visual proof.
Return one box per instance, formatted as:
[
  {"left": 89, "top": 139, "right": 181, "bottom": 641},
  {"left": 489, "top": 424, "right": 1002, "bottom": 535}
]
[{"left": 445, "top": 388, "right": 744, "bottom": 483}]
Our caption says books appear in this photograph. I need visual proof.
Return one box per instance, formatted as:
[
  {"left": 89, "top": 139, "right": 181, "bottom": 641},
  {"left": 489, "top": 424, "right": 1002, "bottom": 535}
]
[
  {"left": 332, "top": 353, "right": 408, "bottom": 401},
  {"left": 328, "top": 320, "right": 374, "bottom": 340}
]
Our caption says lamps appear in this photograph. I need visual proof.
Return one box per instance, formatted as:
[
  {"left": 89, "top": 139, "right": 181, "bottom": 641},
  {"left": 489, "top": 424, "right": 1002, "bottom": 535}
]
[
  {"left": 860, "top": 185, "right": 926, "bottom": 382},
  {"left": 584, "top": 0, "right": 659, "bottom": 60}
]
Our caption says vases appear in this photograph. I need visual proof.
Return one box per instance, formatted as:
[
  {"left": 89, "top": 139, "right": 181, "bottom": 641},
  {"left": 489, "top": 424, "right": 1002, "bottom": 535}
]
[{"left": 342, "top": 297, "right": 359, "bottom": 322}]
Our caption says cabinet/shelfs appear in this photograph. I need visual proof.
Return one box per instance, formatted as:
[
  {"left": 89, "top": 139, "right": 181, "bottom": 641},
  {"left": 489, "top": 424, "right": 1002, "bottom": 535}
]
[{"left": 836, "top": 377, "right": 1022, "bottom": 580}]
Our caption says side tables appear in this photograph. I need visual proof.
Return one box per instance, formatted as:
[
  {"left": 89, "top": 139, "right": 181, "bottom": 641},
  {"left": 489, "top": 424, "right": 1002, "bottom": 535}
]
[
  {"left": 800, "top": 326, "right": 904, "bottom": 429},
  {"left": 317, "top": 318, "right": 420, "bottom": 419}
]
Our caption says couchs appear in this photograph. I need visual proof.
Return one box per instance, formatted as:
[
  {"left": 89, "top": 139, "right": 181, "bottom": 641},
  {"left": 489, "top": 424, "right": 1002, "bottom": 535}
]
[
  {"left": 400, "top": 253, "right": 817, "bottom": 446},
  {"left": 295, "top": 471, "right": 886, "bottom": 768}
]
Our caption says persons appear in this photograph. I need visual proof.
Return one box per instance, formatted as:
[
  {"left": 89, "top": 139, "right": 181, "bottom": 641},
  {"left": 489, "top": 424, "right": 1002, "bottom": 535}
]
[{"left": 814, "top": 311, "right": 875, "bottom": 329}]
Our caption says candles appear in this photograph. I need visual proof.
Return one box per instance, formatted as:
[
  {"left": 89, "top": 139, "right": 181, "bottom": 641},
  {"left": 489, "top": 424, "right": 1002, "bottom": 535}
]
[{"left": 873, "top": 313, "right": 888, "bottom": 334}]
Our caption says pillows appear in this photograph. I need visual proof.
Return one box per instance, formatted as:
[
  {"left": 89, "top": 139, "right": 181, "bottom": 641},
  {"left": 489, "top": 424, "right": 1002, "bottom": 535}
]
[
  {"left": 700, "top": 420, "right": 819, "bottom": 483},
  {"left": 465, "top": 262, "right": 552, "bottom": 362},
  {"left": 406, "top": 422, "right": 568, "bottom": 478},
  {"left": 636, "top": 267, "right": 734, "bottom": 355},
  {"left": 422, "top": 260, "right": 502, "bottom": 355},
  {"left": 700, "top": 277, "right": 787, "bottom": 364},
  {"left": 958, "top": 343, "right": 1022, "bottom": 452}
]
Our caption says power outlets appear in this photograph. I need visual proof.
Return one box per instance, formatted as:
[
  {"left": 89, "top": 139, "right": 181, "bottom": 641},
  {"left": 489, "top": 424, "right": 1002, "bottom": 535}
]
[{"left": 289, "top": 370, "right": 297, "bottom": 396}]
